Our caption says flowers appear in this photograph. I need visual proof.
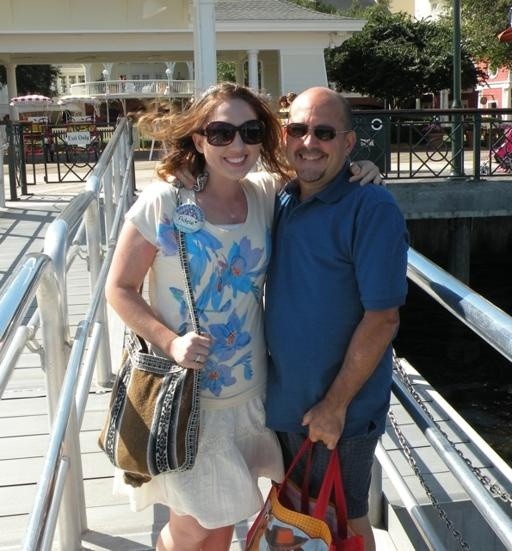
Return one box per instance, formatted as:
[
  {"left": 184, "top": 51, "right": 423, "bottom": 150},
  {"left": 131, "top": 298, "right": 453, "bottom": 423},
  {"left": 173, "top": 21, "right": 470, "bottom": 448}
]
[{"left": 423, "top": 123, "right": 443, "bottom": 134}]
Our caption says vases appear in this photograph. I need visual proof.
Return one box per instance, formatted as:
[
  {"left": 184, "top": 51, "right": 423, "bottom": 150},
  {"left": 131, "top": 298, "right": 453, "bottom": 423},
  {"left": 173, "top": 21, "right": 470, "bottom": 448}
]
[{"left": 428, "top": 133, "right": 443, "bottom": 149}]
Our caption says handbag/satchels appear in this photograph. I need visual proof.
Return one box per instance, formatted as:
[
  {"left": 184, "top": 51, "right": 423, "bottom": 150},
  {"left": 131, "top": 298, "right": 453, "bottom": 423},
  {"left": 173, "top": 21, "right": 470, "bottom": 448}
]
[
  {"left": 244, "top": 436, "right": 365, "bottom": 551},
  {"left": 96, "top": 328, "right": 203, "bottom": 490}
]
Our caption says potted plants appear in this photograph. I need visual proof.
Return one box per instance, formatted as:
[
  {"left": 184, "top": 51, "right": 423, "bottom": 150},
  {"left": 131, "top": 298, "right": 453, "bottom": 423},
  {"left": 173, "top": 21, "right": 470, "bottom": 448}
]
[{"left": 462, "top": 123, "right": 482, "bottom": 148}]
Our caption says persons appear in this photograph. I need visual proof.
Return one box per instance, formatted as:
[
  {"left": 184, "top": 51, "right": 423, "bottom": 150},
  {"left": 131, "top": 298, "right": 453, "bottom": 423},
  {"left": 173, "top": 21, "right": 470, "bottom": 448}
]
[
  {"left": 286, "top": 92, "right": 297, "bottom": 105},
  {"left": 260, "top": 85, "right": 411, "bottom": 551},
  {"left": 101, "top": 80, "right": 387, "bottom": 551},
  {"left": 275, "top": 95, "right": 289, "bottom": 127}
]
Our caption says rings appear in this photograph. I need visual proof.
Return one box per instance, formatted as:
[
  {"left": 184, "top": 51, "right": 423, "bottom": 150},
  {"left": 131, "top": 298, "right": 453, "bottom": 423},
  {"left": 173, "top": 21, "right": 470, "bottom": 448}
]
[{"left": 193, "top": 354, "right": 201, "bottom": 363}]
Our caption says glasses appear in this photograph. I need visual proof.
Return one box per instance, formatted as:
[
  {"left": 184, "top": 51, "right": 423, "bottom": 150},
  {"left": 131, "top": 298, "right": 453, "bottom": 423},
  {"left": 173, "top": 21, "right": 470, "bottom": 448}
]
[
  {"left": 194, "top": 118, "right": 266, "bottom": 147},
  {"left": 284, "top": 122, "right": 353, "bottom": 142}
]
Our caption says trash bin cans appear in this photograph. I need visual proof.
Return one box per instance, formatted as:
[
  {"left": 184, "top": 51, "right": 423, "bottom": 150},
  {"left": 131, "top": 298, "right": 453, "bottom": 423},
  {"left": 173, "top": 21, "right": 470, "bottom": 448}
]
[{"left": 346, "top": 96, "right": 391, "bottom": 179}]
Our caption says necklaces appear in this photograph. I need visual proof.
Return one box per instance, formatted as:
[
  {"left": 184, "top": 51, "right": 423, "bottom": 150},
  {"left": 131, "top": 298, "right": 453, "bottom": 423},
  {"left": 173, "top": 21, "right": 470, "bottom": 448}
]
[{"left": 196, "top": 184, "right": 244, "bottom": 224}]
